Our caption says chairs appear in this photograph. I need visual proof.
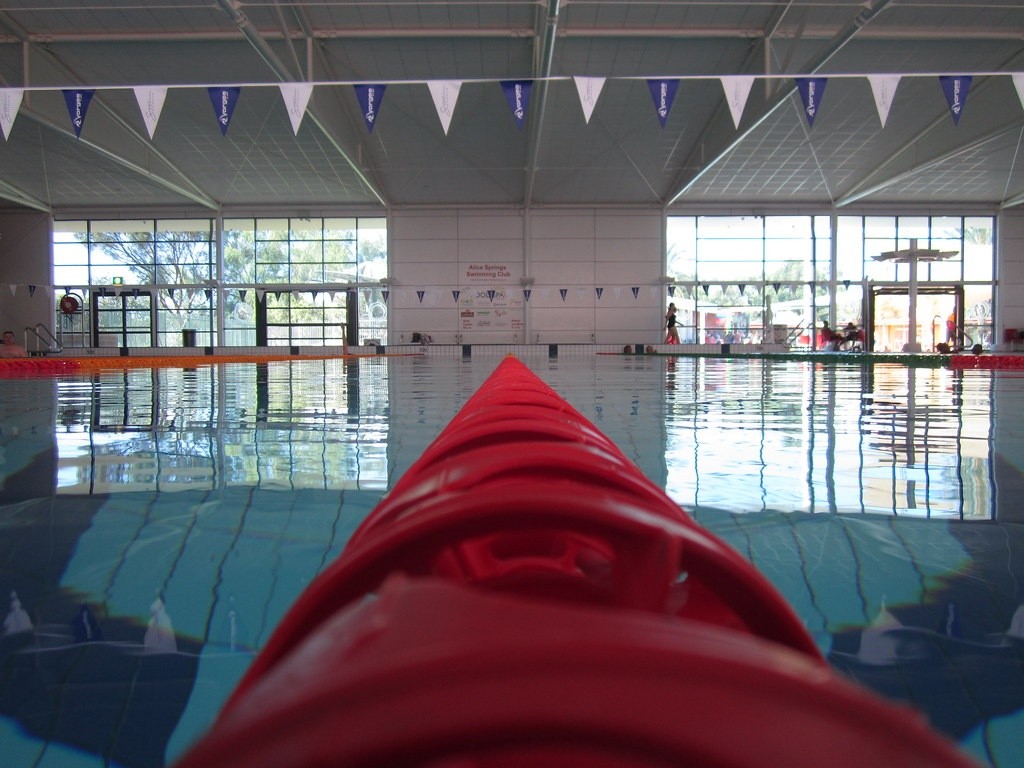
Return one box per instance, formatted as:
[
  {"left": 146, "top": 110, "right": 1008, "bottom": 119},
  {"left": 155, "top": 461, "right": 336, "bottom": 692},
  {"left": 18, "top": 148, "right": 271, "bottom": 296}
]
[
  {"left": 821, "top": 328, "right": 844, "bottom": 352},
  {"left": 853, "top": 327, "right": 866, "bottom": 352}
]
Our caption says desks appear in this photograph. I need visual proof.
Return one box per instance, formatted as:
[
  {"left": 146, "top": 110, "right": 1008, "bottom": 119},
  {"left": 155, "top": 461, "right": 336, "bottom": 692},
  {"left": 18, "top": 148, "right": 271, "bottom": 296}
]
[{"left": 834, "top": 330, "right": 858, "bottom": 352}]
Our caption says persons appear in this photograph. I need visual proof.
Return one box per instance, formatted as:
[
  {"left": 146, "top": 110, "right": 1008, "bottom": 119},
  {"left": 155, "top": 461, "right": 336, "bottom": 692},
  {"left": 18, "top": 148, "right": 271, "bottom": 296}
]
[
  {"left": 623, "top": 345, "right": 632, "bottom": 354},
  {"left": 946, "top": 307, "right": 956, "bottom": 346},
  {"left": 646, "top": 346, "right": 654, "bottom": 353},
  {"left": 662, "top": 303, "right": 683, "bottom": 344},
  {"left": 822, "top": 321, "right": 845, "bottom": 350},
  {"left": 669, "top": 307, "right": 678, "bottom": 345},
  {"left": 843, "top": 323, "right": 858, "bottom": 349},
  {"left": 0, "top": 330, "right": 28, "bottom": 358}
]
